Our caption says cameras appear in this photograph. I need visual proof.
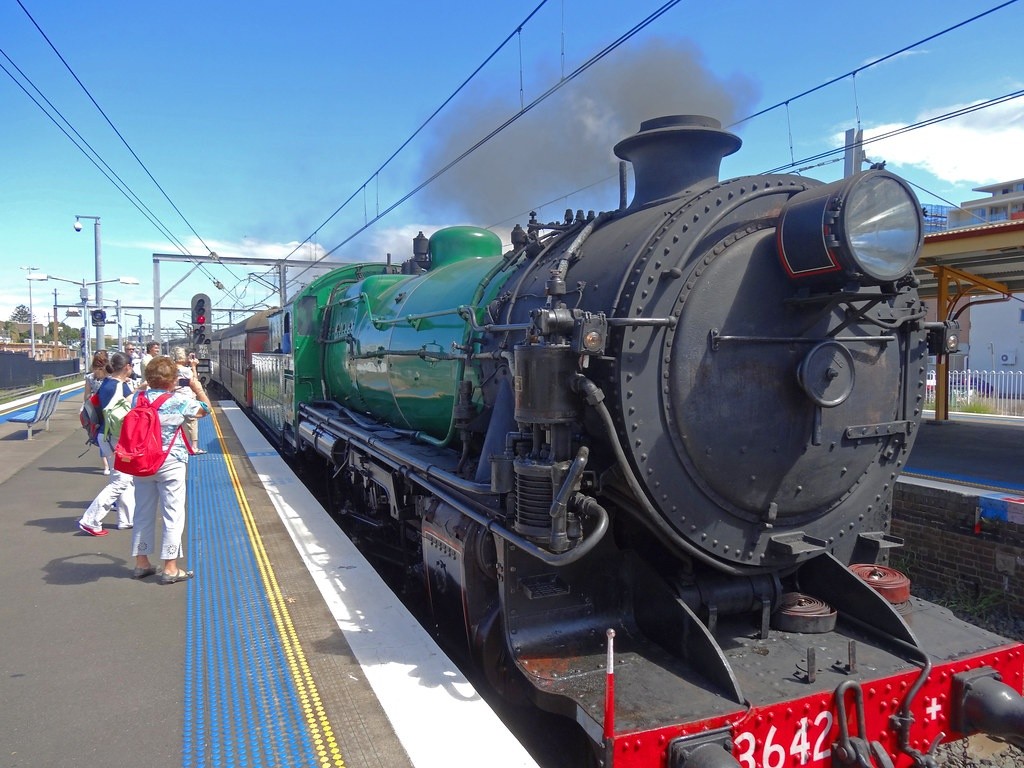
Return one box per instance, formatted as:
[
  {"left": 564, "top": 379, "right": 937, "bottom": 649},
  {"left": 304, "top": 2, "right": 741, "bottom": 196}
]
[
  {"left": 179, "top": 379, "right": 190, "bottom": 386},
  {"left": 191, "top": 354, "right": 195, "bottom": 360}
]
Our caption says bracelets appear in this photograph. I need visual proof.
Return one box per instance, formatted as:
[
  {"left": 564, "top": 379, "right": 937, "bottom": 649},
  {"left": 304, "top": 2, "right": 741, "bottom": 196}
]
[{"left": 196, "top": 389, "right": 203, "bottom": 394}]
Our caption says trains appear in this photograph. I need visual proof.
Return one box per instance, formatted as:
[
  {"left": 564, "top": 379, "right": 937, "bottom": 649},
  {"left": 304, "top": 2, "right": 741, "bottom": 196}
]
[{"left": 160, "top": 115, "right": 1021, "bottom": 768}]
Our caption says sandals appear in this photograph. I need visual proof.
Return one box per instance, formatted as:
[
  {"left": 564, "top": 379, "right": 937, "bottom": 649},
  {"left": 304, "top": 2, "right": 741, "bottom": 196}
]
[
  {"left": 161, "top": 568, "right": 194, "bottom": 585},
  {"left": 134, "top": 565, "right": 164, "bottom": 578}
]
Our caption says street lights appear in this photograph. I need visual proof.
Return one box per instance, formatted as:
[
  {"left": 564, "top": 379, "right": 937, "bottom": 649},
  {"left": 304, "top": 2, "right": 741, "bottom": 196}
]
[
  {"left": 20, "top": 265, "right": 40, "bottom": 358},
  {"left": 75, "top": 297, "right": 123, "bottom": 353},
  {"left": 111, "top": 312, "right": 142, "bottom": 359},
  {"left": 73, "top": 214, "right": 106, "bottom": 360},
  {"left": 26, "top": 274, "right": 139, "bottom": 378}
]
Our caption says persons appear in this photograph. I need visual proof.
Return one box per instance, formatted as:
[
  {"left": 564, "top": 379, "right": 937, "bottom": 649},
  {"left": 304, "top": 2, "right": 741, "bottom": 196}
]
[
  {"left": 131, "top": 356, "right": 213, "bottom": 584},
  {"left": 78, "top": 341, "right": 208, "bottom": 537}
]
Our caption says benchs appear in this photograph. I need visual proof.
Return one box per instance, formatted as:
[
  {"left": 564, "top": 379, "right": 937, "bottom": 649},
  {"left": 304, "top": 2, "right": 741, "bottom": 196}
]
[{"left": 6, "top": 390, "right": 61, "bottom": 441}]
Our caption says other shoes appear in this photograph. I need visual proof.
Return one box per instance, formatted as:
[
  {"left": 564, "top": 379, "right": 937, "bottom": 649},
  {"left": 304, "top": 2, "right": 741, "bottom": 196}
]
[
  {"left": 188, "top": 448, "right": 207, "bottom": 455},
  {"left": 103, "top": 468, "right": 110, "bottom": 475},
  {"left": 79, "top": 523, "right": 109, "bottom": 537}
]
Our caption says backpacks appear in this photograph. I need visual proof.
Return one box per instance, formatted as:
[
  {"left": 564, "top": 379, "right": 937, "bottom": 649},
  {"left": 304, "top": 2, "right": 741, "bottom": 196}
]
[
  {"left": 102, "top": 394, "right": 133, "bottom": 443},
  {"left": 79, "top": 386, "right": 100, "bottom": 443},
  {"left": 114, "top": 390, "right": 183, "bottom": 477}
]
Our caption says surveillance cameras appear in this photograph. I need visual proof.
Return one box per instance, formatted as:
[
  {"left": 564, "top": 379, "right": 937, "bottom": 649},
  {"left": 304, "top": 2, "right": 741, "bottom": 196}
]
[{"left": 74, "top": 223, "right": 82, "bottom": 232}]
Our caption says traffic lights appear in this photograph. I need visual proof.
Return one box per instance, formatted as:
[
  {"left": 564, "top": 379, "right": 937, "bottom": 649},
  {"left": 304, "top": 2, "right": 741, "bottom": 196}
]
[{"left": 191, "top": 293, "right": 212, "bottom": 324}]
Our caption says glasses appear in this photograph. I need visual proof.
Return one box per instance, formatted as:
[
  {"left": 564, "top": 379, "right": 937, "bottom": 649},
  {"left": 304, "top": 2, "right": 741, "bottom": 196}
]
[{"left": 127, "top": 348, "right": 135, "bottom": 350}]
